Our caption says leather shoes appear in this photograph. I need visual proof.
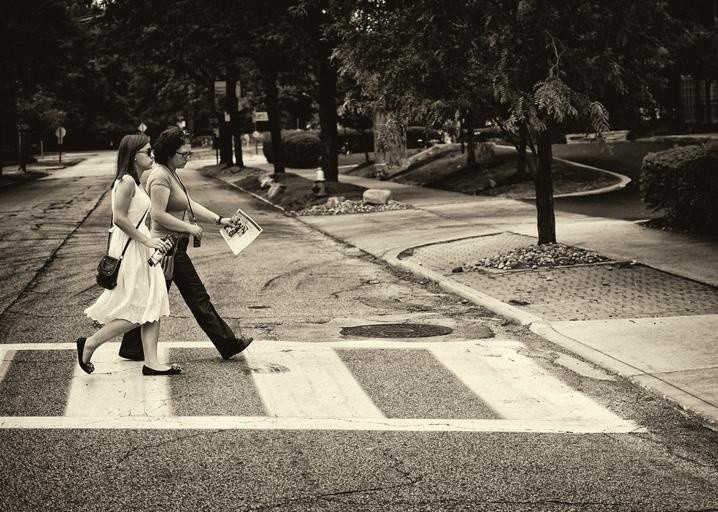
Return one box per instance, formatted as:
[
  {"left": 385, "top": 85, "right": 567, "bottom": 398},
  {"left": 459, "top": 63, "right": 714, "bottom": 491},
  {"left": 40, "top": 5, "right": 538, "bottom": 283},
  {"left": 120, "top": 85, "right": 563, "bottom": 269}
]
[
  {"left": 142, "top": 363, "right": 182, "bottom": 376},
  {"left": 76, "top": 337, "right": 94, "bottom": 374}
]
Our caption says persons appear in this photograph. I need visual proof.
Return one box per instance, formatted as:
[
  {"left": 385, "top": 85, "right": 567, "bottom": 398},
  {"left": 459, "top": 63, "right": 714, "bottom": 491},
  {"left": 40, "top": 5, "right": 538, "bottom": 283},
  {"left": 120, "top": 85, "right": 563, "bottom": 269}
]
[
  {"left": 119, "top": 128, "right": 253, "bottom": 360},
  {"left": 77, "top": 133, "right": 183, "bottom": 376}
]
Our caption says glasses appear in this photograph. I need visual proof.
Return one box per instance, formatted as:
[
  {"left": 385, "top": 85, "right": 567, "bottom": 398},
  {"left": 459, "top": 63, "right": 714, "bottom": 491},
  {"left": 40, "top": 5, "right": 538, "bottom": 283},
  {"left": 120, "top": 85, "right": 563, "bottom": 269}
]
[
  {"left": 136, "top": 148, "right": 152, "bottom": 156},
  {"left": 176, "top": 151, "right": 194, "bottom": 159}
]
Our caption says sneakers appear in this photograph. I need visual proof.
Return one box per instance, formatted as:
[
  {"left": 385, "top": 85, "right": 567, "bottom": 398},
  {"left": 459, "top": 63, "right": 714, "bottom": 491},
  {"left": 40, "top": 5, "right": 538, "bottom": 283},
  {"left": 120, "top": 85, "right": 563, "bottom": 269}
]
[{"left": 225, "top": 337, "right": 253, "bottom": 360}]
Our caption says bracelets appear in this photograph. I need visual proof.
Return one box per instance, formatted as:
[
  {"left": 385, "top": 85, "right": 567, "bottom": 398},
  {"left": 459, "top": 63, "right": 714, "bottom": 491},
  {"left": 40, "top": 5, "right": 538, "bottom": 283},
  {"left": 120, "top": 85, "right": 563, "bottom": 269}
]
[{"left": 218, "top": 216, "right": 223, "bottom": 225}]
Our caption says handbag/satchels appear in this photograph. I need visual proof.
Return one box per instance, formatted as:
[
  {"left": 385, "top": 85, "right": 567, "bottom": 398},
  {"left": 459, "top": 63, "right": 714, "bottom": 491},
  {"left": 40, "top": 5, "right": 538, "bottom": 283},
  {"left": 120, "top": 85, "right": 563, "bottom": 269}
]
[
  {"left": 159, "top": 255, "right": 176, "bottom": 281},
  {"left": 96, "top": 255, "right": 123, "bottom": 291}
]
